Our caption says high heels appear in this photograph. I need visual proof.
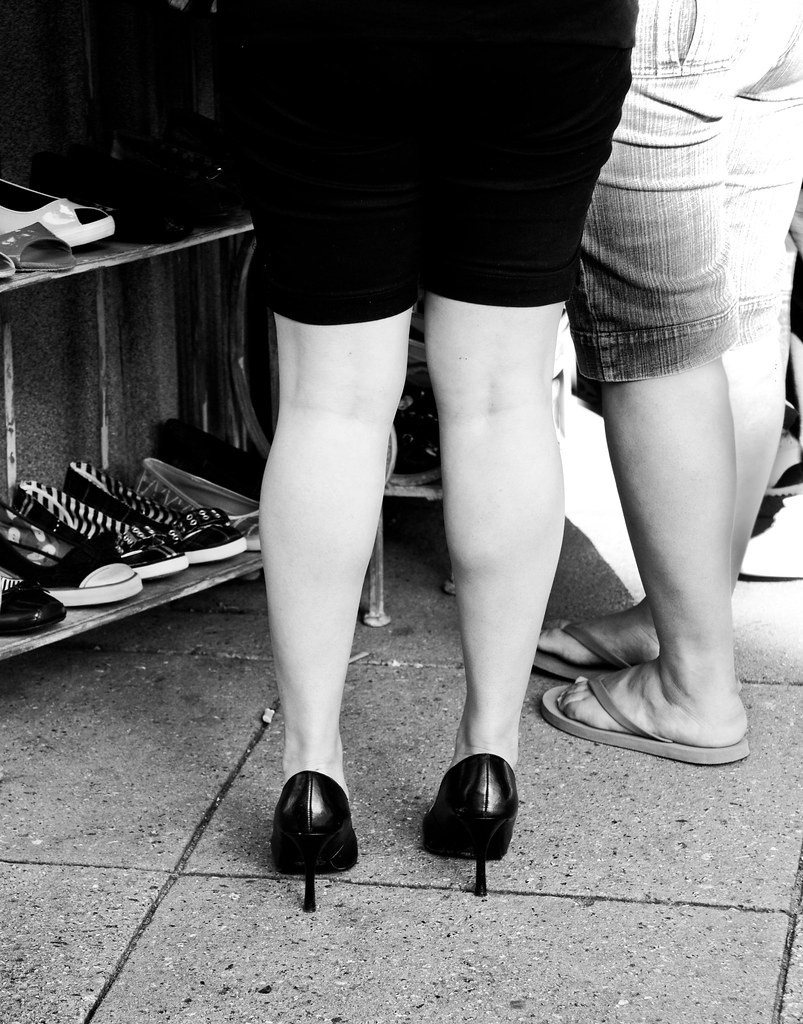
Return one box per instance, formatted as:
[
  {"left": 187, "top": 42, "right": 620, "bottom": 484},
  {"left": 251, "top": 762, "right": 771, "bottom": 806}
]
[
  {"left": 422, "top": 753, "right": 519, "bottom": 896},
  {"left": 271, "top": 771, "right": 358, "bottom": 912}
]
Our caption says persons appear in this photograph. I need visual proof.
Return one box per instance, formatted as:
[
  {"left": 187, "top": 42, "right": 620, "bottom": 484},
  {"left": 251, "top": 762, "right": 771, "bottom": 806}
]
[
  {"left": 529, "top": 1, "right": 802, "bottom": 766},
  {"left": 258, "top": 1, "right": 638, "bottom": 912}
]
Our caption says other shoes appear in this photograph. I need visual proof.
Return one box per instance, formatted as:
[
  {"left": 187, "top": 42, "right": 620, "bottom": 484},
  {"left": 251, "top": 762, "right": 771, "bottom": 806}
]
[
  {"left": 1, "top": 116, "right": 243, "bottom": 292},
  {"left": 0, "top": 415, "right": 261, "bottom": 636}
]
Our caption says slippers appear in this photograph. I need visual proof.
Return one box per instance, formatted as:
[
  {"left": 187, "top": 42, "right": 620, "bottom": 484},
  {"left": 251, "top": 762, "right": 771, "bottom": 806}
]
[
  {"left": 540, "top": 672, "right": 749, "bottom": 765},
  {"left": 533, "top": 620, "right": 631, "bottom": 680}
]
